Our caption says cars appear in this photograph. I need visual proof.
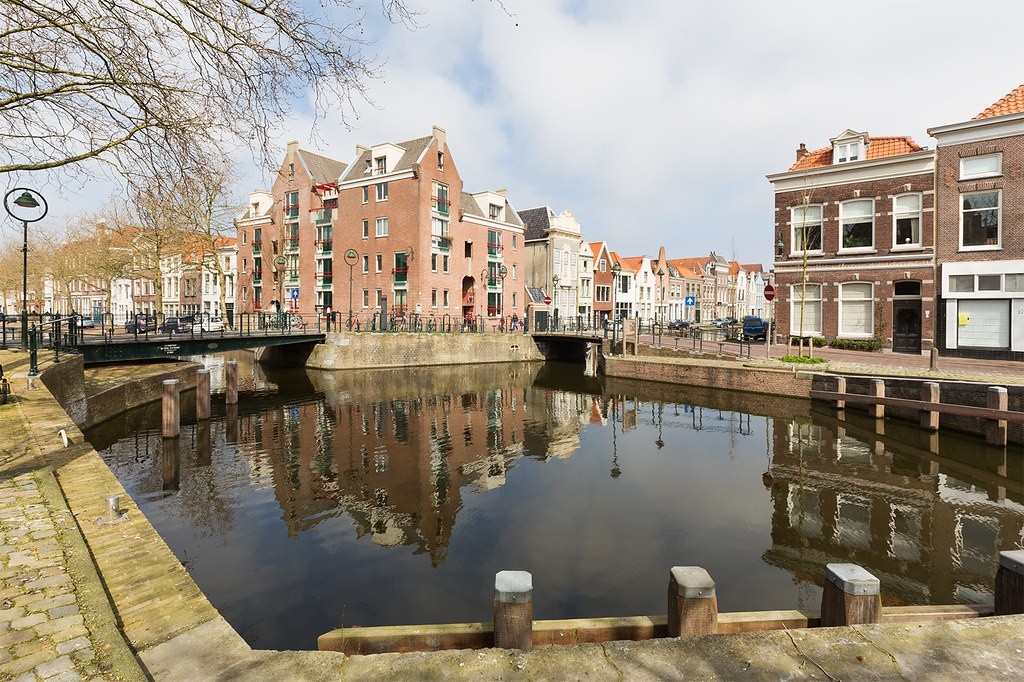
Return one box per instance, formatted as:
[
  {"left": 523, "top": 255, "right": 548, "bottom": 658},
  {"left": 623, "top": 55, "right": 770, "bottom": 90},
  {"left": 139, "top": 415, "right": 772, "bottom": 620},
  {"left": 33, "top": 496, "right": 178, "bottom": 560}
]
[
  {"left": 160, "top": 316, "right": 196, "bottom": 335},
  {"left": 77, "top": 316, "right": 95, "bottom": 328},
  {"left": 125, "top": 316, "right": 156, "bottom": 333},
  {"left": 726, "top": 317, "right": 738, "bottom": 326},
  {"left": 190, "top": 317, "right": 225, "bottom": 334},
  {"left": 667, "top": 322, "right": 689, "bottom": 331},
  {"left": 602, "top": 319, "right": 624, "bottom": 331},
  {"left": 712, "top": 318, "right": 730, "bottom": 327}
]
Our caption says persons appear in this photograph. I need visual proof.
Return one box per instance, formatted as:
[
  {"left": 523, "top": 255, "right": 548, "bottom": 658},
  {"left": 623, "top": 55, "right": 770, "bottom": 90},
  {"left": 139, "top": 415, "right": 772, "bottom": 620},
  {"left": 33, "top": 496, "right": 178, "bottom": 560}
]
[
  {"left": 518, "top": 318, "right": 524, "bottom": 330},
  {"left": 512, "top": 312, "right": 519, "bottom": 330}
]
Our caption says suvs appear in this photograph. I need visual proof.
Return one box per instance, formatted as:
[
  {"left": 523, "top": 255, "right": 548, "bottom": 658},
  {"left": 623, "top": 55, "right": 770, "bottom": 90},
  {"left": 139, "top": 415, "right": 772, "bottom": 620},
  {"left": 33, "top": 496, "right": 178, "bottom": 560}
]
[{"left": 742, "top": 316, "right": 775, "bottom": 341}]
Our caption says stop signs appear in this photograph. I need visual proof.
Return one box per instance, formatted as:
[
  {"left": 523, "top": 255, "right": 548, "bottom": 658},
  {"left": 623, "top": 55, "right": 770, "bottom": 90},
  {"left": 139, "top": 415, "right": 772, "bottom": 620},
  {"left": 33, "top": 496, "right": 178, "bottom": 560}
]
[
  {"left": 764, "top": 285, "right": 775, "bottom": 301},
  {"left": 544, "top": 297, "right": 552, "bottom": 305}
]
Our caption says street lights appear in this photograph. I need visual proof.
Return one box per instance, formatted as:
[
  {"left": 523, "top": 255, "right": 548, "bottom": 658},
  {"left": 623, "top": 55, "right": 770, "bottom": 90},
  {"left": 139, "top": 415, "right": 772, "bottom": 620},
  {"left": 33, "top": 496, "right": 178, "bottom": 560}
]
[
  {"left": 344, "top": 249, "right": 359, "bottom": 332},
  {"left": 499, "top": 265, "right": 507, "bottom": 333},
  {"left": 3, "top": 188, "right": 48, "bottom": 352},
  {"left": 657, "top": 267, "right": 665, "bottom": 336},
  {"left": 274, "top": 256, "right": 288, "bottom": 329},
  {"left": 610, "top": 261, "right": 622, "bottom": 331},
  {"left": 552, "top": 274, "right": 559, "bottom": 331}
]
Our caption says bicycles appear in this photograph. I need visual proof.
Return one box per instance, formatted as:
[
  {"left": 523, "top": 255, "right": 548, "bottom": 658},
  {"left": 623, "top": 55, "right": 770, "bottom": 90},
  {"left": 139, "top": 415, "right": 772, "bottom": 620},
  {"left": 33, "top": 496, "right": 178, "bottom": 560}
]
[
  {"left": 345, "top": 310, "right": 362, "bottom": 332},
  {"left": 259, "top": 310, "right": 303, "bottom": 329},
  {"left": 371, "top": 309, "right": 438, "bottom": 332}
]
